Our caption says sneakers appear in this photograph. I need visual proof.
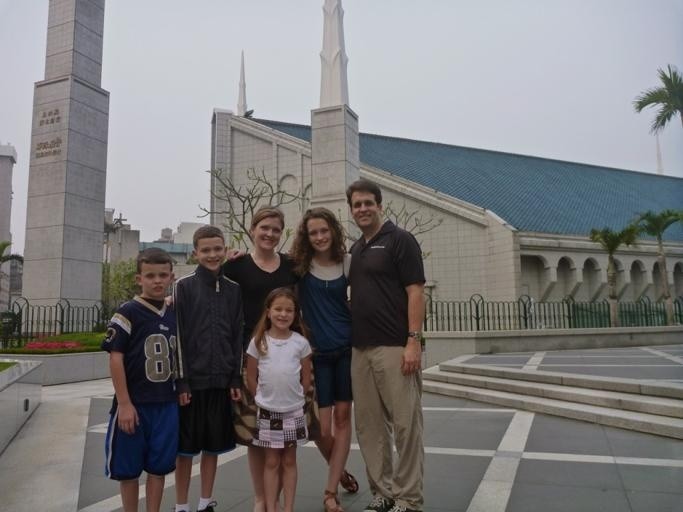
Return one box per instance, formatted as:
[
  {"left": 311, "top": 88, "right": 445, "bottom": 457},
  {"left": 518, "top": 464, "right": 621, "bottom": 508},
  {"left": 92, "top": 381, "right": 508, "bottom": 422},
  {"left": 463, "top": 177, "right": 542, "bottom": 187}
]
[
  {"left": 363, "top": 497, "right": 391, "bottom": 510},
  {"left": 197, "top": 504, "right": 218, "bottom": 512},
  {"left": 390, "top": 505, "right": 419, "bottom": 512}
]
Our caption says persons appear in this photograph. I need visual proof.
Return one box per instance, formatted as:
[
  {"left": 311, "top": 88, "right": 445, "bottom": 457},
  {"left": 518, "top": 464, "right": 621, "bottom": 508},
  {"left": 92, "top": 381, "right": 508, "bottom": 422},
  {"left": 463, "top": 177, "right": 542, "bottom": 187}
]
[
  {"left": 245, "top": 286, "right": 312, "bottom": 512},
  {"left": 345, "top": 180, "right": 426, "bottom": 512},
  {"left": 101, "top": 247, "right": 180, "bottom": 512},
  {"left": 226, "top": 207, "right": 359, "bottom": 511},
  {"left": 173, "top": 226, "right": 243, "bottom": 511},
  {"left": 163, "top": 204, "right": 295, "bottom": 512}
]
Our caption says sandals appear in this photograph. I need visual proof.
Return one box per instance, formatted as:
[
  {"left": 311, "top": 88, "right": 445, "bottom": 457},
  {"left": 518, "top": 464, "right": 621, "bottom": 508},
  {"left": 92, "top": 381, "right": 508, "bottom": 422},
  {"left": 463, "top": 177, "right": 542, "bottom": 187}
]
[
  {"left": 320, "top": 491, "right": 341, "bottom": 510},
  {"left": 339, "top": 471, "right": 359, "bottom": 493}
]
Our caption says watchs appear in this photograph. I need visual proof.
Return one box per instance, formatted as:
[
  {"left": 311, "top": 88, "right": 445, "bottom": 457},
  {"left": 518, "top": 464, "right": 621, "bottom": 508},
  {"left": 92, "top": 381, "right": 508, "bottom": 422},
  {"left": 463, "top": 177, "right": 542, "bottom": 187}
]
[{"left": 408, "top": 331, "right": 423, "bottom": 342}]
